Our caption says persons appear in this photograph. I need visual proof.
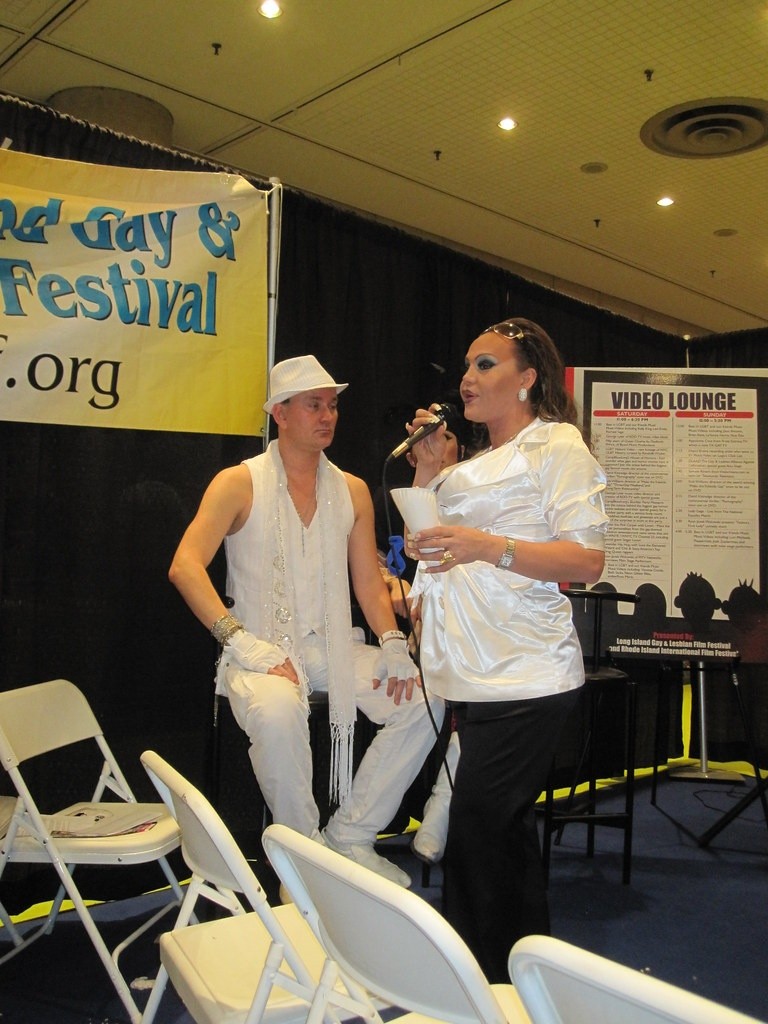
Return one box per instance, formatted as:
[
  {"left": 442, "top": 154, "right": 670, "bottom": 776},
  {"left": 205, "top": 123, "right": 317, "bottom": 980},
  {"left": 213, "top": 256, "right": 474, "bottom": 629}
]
[
  {"left": 170, "top": 352, "right": 446, "bottom": 905},
  {"left": 403, "top": 318, "right": 608, "bottom": 985},
  {"left": 373, "top": 403, "right": 467, "bottom": 864}
]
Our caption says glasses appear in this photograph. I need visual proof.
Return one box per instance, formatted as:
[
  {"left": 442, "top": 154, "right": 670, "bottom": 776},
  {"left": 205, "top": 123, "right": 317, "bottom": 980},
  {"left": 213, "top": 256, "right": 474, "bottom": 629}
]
[{"left": 478, "top": 322, "right": 526, "bottom": 340}]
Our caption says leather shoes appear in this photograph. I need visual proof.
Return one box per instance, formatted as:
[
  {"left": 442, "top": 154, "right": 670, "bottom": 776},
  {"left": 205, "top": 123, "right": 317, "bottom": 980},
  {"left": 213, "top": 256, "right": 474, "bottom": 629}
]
[
  {"left": 319, "top": 828, "right": 412, "bottom": 889},
  {"left": 277, "top": 883, "right": 291, "bottom": 904}
]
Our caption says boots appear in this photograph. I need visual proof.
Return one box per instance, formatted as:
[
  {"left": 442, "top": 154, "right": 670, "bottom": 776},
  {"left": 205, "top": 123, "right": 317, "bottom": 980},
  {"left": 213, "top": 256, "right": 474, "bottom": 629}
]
[{"left": 410, "top": 731, "right": 469, "bottom": 864}]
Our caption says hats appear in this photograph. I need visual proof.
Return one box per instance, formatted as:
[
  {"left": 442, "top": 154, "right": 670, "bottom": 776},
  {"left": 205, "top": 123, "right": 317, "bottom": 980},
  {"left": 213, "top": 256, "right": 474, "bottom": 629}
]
[{"left": 263, "top": 355, "right": 349, "bottom": 414}]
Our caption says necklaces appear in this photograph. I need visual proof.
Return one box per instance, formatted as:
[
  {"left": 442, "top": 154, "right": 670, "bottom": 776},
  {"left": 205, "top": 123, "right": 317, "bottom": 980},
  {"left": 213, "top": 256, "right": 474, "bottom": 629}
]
[
  {"left": 287, "top": 482, "right": 316, "bottom": 559},
  {"left": 502, "top": 433, "right": 517, "bottom": 446}
]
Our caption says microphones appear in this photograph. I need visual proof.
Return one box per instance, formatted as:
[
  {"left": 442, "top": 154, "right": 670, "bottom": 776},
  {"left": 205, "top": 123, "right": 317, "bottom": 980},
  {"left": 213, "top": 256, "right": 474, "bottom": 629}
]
[{"left": 386, "top": 403, "right": 457, "bottom": 466}]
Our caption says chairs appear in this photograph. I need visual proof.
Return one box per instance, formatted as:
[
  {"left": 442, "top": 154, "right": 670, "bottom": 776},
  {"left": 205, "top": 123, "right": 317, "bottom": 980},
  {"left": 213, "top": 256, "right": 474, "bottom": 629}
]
[
  {"left": 530, "top": 588, "right": 639, "bottom": 890},
  {"left": 214, "top": 589, "right": 431, "bottom": 891},
  {"left": 0, "top": 679, "right": 768, "bottom": 1024}
]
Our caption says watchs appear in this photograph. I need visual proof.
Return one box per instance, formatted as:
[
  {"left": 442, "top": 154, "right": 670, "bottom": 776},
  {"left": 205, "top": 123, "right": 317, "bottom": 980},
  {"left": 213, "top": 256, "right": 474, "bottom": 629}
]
[{"left": 495, "top": 536, "right": 515, "bottom": 569}]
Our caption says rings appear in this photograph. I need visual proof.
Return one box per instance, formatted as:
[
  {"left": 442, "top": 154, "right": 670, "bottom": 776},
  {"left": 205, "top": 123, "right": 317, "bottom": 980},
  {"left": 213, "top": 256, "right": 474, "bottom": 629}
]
[{"left": 442, "top": 550, "right": 456, "bottom": 565}]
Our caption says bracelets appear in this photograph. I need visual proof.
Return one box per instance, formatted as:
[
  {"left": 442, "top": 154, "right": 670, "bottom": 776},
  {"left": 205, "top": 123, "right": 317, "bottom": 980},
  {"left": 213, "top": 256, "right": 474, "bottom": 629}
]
[
  {"left": 210, "top": 614, "right": 243, "bottom": 644},
  {"left": 379, "top": 630, "right": 406, "bottom": 648}
]
